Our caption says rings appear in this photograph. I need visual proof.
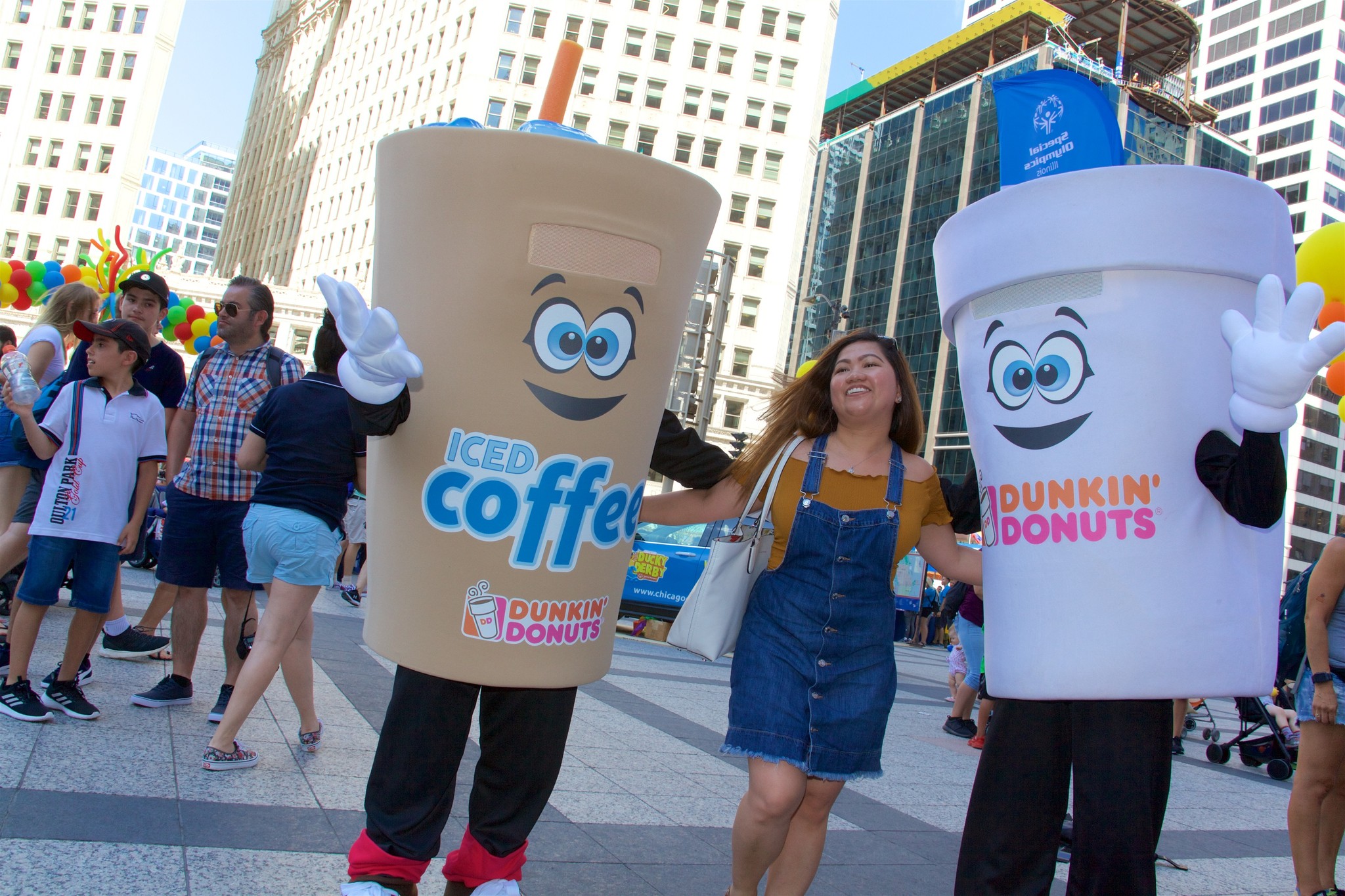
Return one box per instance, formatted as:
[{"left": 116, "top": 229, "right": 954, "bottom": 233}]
[{"left": 1315, "top": 714, "right": 1321, "bottom": 717}]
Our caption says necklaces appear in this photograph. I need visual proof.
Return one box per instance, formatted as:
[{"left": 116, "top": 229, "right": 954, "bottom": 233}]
[{"left": 834, "top": 430, "right": 889, "bottom": 474}]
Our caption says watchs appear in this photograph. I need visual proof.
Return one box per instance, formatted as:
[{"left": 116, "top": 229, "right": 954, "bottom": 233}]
[{"left": 1311, "top": 672, "right": 1333, "bottom": 684}]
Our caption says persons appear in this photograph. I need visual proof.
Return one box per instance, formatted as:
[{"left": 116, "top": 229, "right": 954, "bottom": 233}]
[
  {"left": 324, "top": 480, "right": 368, "bottom": 606},
  {"left": 199, "top": 307, "right": 367, "bottom": 771},
  {"left": 938, "top": 273, "right": 1345, "bottom": 896},
  {"left": 1286, "top": 515, "right": 1345, "bottom": 896},
  {"left": 0, "top": 316, "right": 169, "bottom": 723},
  {"left": 1172, "top": 698, "right": 1189, "bottom": 754},
  {"left": 317, "top": 271, "right": 739, "bottom": 894},
  {"left": 639, "top": 325, "right": 983, "bottom": 896},
  {"left": 130, "top": 274, "right": 305, "bottom": 724},
  {"left": 941, "top": 548, "right": 995, "bottom": 749},
  {"left": 893, "top": 576, "right": 957, "bottom": 649},
  {"left": 0, "top": 271, "right": 187, "bottom": 689},
  {"left": 1258, "top": 686, "right": 1300, "bottom": 748}
]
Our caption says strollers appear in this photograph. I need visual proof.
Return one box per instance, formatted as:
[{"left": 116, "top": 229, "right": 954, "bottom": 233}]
[
  {"left": 1205, "top": 677, "right": 1300, "bottom": 781},
  {"left": 1179, "top": 698, "right": 1220, "bottom": 744}
]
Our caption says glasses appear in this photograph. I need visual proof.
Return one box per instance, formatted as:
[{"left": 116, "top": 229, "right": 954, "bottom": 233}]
[
  {"left": 214, "top": 302, "right": 255, "bottom": 318},
  {"left": 878, "top": 335, "right": 899, "bottom": 352}
]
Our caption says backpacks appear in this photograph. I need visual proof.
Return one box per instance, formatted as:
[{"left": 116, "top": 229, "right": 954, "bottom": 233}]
[
  {"left": 1276, "top": 533, "right": 1345, "bottom": 691},
  {"left": 927, "top": 588, "right": 940, "bottom": 612},
  {"left": 944, "top": 580, "right": 969, "bottom": 621}
]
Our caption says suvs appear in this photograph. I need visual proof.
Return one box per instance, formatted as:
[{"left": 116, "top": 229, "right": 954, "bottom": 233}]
[{"left": 618, "top": 511, "right": 776, "bottom": 624}]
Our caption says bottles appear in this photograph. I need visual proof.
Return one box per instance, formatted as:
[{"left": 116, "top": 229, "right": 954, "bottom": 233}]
[{"left": 0, "top": 345, "right": 41, "bottom": 406}]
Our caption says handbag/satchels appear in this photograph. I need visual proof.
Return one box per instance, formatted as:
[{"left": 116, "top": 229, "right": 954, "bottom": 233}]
[
  {"left": 665, "top": 432, "right": 807, "bottom": 665},
  {"left": 352, "top": 552, "right": 362, "bottom": 574}
]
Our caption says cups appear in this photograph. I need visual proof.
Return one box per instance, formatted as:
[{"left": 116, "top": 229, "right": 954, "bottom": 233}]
[
  {"left": 929, "top": 165, "right": 1298, "bottom": 701},
  {"left": 362, "top": 126, "right": 723, "bottom": 690}
]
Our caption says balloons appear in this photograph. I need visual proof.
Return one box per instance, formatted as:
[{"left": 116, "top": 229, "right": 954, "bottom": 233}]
[
  {"left": 1328, "top": 351, "right": 1345, "bottom": 365},
  {"left": 78, "top": 223, "right": 172, "bottom": 324},
  {"left": 0, "top": 260, "right": 108, "bottom": 311},
  {"left": 158, "top": 292, "right": 224, "bottom": 355},
  {"left": 1295, "top": 221, "right": 1345, "bottom": 308},
  {"left": 1326, "top": 361, "right": 1345, "bottom": 397},
  {"left": 1318, "top": 301, "right": 1345, "bottom": 331},
  {"left": 1338, "top": 395, "right": 1345, "bottom": 424}
]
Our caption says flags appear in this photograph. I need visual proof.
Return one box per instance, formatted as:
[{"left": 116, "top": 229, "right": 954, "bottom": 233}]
[{"left": 993, "top": 69, "right": 1125, "bottom": 194}]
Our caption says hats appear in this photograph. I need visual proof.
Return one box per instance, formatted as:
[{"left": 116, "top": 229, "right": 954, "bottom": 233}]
[
  {"left": 118, "top": 271, "right": 170, "bottom": 308},
  {"left": 72, "top": 318, "right": 152, "bottom": 363}
]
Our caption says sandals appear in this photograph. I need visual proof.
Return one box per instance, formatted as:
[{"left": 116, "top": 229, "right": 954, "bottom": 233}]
[
  {"left": 147, "top": 648, "right": 172, "bottom": 660},
  {"left": 0, "top": 593, "right": 13, "bottom": 616},
  {"left": 905, "top": 640, "right": 926, "bottom": 647}
]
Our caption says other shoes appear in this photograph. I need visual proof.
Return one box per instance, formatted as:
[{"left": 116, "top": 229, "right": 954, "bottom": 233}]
[
  {"left": 902, "top": 637, "right": 912, "bottom": 642},
  {"left": 944, "top": 696, "right": 955, "bottom": 702}
]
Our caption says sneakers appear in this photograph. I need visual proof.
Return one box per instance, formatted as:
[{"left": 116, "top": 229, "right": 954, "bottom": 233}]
[
  {"left": 298, "top": 717, "right": 324, "bottom": 753},
  {"left": 942, "top": 715, "right": 977, "bottom": 738},
  {"left": 130, "top": 673, "right": 193, "bottom": 708},
  {"left": 0, "top": 676, "right": 55, "bottom": 721},
  {"left": 340, "top": 585, "right": 361, "bottom": 606},
  {"left": 1172, "top": 735, "right": 1185, "bottom": 754},
  {"left": 932, "top": 643, "right": 945, "bottom": 648},
  {"left": 1284, "top": 734, "right": 1300, "bottom": 748},
  {"left": 326, "top": 581, "right": 356, "bottom": 592},
  {"left": 208, "top": 685, "right": 234, "bottom": 722},
  {"left": 967, "top": 732, "right": 986, "bottom": 749},
  {"left": 201, "top": 741, "right": 260, "bottom": 770},
  {"left": 97, "top": 626, "right": 171, "bottom": 658},
  {"left": 1294, "top": 883, "right": 1345, "bottom": 896},
  {"left": 0, "top": 642, "right": 93, "bottom": 689},
  {"left": 39, "top": 677, "right": 101, "bottom": 720}
]
